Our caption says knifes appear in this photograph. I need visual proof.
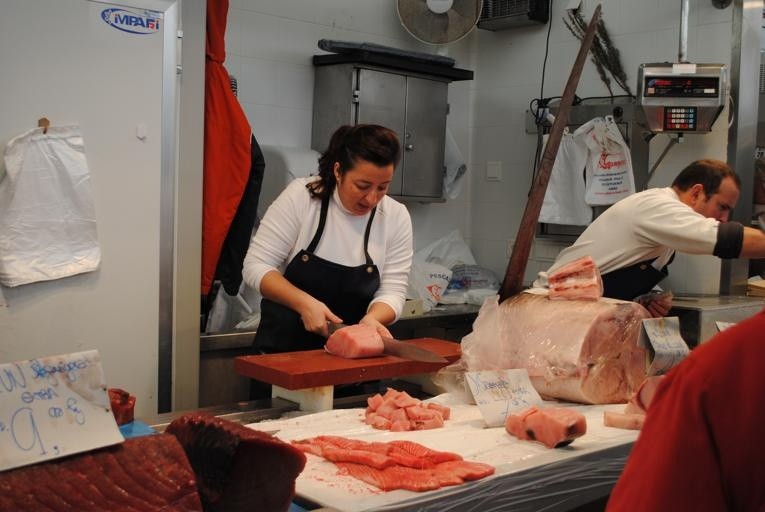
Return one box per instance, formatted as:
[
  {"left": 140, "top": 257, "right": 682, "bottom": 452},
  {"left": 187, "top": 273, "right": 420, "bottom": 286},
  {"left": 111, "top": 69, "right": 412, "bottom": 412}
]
[{"left": 317, "top": 318, "right": 451, "bottom": 364}]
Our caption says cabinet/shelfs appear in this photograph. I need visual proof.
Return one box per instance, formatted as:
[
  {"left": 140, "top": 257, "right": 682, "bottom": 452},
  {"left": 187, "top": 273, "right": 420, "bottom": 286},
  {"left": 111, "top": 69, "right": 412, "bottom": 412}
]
[{"left": 311, "top": 63, "right": 453, "bottom": 203}]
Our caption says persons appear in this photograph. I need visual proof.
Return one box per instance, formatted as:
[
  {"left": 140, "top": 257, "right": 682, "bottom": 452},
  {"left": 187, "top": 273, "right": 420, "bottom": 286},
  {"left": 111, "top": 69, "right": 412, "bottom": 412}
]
[
  {"left": 238, "top": 123, "right": 416, "bottom": 401},
  {"left": 527, "top": 157, "right": 765, "bottom": 319},
  {"left": 604, "top": 307, "right": 765, "bottom": 512}
]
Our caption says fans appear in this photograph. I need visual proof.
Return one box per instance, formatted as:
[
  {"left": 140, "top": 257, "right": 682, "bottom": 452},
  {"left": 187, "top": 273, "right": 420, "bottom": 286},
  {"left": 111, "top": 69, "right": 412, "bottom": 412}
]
[{"left": 395, "top": 0, "right": 485, "bottom": 47}]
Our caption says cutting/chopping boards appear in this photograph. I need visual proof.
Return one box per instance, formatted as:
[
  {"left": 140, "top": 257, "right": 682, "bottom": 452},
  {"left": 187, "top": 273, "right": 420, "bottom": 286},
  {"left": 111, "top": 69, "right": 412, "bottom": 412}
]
[{"left": 234, "top": 335, "right": 463, "bottom": 389}]
